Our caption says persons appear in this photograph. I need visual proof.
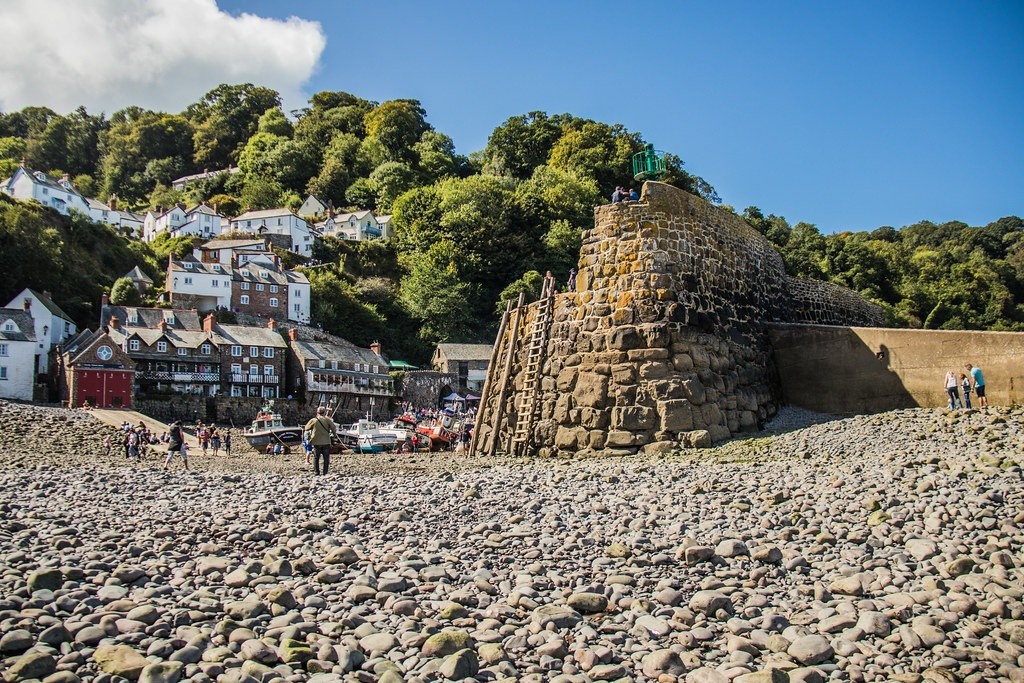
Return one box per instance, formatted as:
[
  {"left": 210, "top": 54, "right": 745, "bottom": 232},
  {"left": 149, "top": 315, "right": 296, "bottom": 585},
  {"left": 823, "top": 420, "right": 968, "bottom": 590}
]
[
  {"left": 210, "top": 389, "right": 222, "bottom": 397},
  {"left": 545, "top": 268, "right": 577, "bottom": 296},
  {"left": 256, "top": 397, "right": 272, "bottom": 420},
  {"left": 392, "top": 400, "right": 479, "bottom": 455},
  {"left": 104, "top": 419, "right": 284, "bottom": 460},
  {"left": 164, "top": 420, "right": 190, "bottom": 470},
  {"left": 83, "top": 400, "right": 90, "bottom": 411},
  {"left": 305, "top": 406, "right": 336, "bottom": 476},
  {"left": 612, "top": 185, "right": 639, "bottom": 202},
  {"left": 944, "top": 362, "right": 989, "bottom": 411}
]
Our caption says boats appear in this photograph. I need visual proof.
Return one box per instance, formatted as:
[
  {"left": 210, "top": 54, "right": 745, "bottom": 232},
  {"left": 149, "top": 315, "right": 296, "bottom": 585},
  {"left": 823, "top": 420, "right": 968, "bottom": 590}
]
[
  {"left": 243, "top": 406, "right": 303, "bottom": 454},
  {"left": 317, "top": 398, "right": 460, "bottom": 453}
]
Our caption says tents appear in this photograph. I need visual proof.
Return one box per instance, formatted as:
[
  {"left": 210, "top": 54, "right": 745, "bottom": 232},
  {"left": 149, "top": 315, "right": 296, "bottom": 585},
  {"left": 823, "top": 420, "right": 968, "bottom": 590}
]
[
  {"left": 388, "top": 360, "right": 420, "bottom": 369},
  {"left": 442, "top": 391, "right": 481, "bottom": 412}
]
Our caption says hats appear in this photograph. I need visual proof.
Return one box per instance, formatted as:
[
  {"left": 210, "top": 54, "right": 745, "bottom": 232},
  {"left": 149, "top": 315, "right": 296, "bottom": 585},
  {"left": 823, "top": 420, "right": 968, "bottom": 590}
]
[{"left": 316, "top": 407, "right": 325, "bottom": 414}]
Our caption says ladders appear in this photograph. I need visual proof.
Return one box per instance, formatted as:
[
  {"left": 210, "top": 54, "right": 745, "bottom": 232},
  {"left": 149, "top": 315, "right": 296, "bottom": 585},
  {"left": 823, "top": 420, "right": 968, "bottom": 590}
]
[{"left": 512, "top": 277, "right": 556, "bottom": 456}]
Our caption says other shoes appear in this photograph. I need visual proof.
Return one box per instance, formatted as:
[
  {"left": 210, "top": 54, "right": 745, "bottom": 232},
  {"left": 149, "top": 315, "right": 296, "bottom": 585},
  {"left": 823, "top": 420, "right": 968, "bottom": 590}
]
[
  {"left": 305, "top": 462, "right": 312, "bottom": 465},
  {"left": 314, "top": 471, "right": 327, "bottom": 476},
  {"left": 163, "top": 467, "right": 169, "bottom": 471}
]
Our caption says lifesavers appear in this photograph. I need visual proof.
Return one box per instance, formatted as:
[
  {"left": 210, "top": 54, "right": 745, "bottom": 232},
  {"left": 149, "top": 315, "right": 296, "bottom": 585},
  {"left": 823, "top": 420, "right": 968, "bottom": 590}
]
[{"left": 431, "top": 419, "right": 436, "bottom": 427}]
